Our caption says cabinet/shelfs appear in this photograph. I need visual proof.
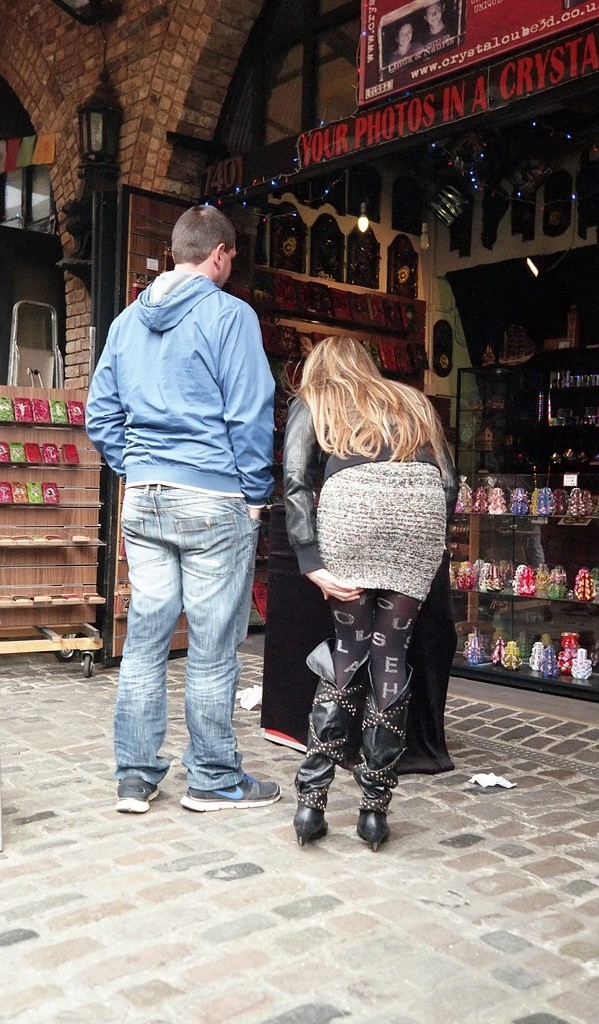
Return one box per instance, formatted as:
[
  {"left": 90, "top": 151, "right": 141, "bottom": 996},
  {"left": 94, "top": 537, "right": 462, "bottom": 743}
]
[
  {"left": 452, "top": 473, "right": 598, "bottom": 705},
  {"left": 0, "top": 387, "right": 107, "bottom": 678},
  {"left": 261, "top": 305, "right": 415, "bottom": 381}
]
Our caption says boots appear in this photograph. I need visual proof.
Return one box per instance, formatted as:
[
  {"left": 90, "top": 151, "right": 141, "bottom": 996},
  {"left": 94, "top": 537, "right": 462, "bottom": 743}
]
[
  {"left": 294, "top": 638, "right": 370, "bottom": 847},
  {"left": 353, "top": 660, "right": 414, "bottom": 852}
]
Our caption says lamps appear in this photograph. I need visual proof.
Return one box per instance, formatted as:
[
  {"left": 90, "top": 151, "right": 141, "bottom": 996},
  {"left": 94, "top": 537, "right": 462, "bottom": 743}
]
[
  {"left": 52, "top": 0, "right": 117, "bottom": 25},
  {"left": 76, "top": 69, "right": 124, "bottom": 166}
]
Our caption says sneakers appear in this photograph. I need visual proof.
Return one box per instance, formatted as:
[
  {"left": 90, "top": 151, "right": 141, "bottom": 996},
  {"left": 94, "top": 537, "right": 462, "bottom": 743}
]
[
  {"left": 116, "top": 773, "right": 159, "bottom": 813},
  {"left": 180, "top": 771, "right": 283, "bottom": 812}
]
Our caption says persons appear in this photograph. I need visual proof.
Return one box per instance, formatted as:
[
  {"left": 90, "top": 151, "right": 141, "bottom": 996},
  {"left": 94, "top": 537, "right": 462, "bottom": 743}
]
[
  {"left": 388, "top": 3, "right": 451, "bottom": 64},
  {"left": 283, "top": 334, "right": 460, "bottom": 849},
  {"left": 85, "top": 205, "right": 281, "bottom": 813}
]
